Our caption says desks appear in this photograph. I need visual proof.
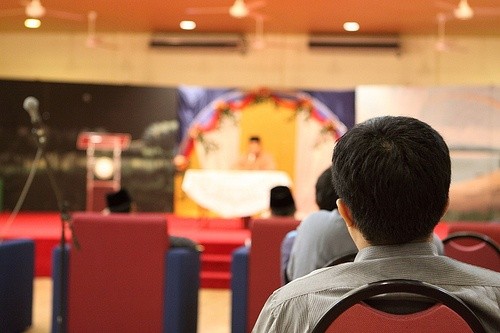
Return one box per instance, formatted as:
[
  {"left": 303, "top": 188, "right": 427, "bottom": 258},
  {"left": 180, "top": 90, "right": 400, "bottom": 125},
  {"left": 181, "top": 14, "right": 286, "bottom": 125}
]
[{"left": 182, "top": 169, "right": 292, "bottom": 229}]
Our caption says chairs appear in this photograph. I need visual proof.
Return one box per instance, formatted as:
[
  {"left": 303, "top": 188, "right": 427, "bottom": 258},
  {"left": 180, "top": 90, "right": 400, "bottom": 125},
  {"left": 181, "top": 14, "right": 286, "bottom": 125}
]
[
  {"left": 232, "top": 217, "right": 301, "bottom": 333},
  {"left": 0, "top": 239, "right": 34, "bottom": 332},
  {"left": 51, "top": 215, "right": 198, "bottom": 332},
  {"left": 441, "top": 231, "right": 500, "bottom": 273},
  {"left": 310, "top": 279, "right": 487, "bottom": 333}
]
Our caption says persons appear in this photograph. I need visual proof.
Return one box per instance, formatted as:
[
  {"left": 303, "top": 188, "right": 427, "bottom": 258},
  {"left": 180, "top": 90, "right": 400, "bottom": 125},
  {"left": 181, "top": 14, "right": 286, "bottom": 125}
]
[
  {"left": 263, "top": 184, "right": 297, "bottom": 219},
  {"left": 240, "top": 136, "right": 276, "bottom": 171},
  {"left": 279, "top": 166, "right": 341, "bottom": 284},
  {"left": 251, "top": 115, "right": 500, "bottom": 333},
  {"left": 100, "top": 191, "right": 199, "bottom": 250},
  {"left": 286, "top": 209, "right": 446, "bottom": 282}
]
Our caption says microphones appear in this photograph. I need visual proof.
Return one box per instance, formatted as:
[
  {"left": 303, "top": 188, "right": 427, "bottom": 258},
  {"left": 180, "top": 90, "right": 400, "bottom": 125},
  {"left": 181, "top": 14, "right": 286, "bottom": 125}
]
[{"left": 23, "top": 97, "right": 47, "bottom": 144}]
